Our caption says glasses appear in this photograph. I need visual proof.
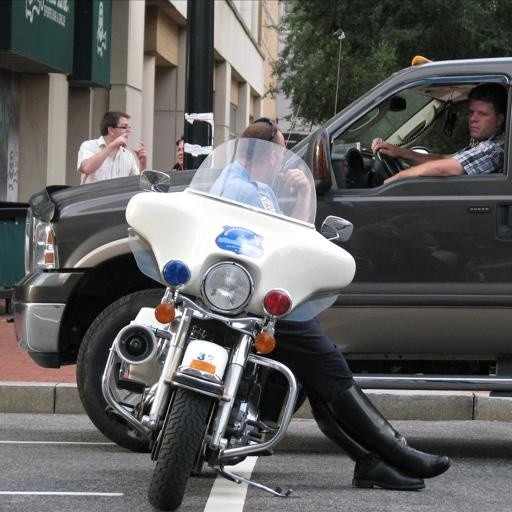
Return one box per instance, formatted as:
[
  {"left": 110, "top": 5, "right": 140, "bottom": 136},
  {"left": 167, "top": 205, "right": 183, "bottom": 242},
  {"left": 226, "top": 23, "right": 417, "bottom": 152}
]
[
  {"left": 112, "top": 124, "right": 131, "bottom": 130},
  {"left": 250, "top": 115, "right": 278, "bottom": 151}
]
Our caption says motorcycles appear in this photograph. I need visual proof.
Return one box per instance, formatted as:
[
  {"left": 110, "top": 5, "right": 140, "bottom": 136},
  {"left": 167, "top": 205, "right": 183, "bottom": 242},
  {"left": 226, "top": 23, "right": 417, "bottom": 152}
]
[{"left": 98, "top": 137, "right": 358, "bottom": 510}]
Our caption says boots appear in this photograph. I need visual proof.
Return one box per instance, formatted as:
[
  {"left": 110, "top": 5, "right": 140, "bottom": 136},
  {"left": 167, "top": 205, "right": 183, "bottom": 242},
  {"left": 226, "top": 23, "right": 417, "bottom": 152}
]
[
  {"left": 311, "top": 399, "right": 427, "bottom": 491},
  {"left": 324, "top": 382, "right": 453, "bottom": 478}
]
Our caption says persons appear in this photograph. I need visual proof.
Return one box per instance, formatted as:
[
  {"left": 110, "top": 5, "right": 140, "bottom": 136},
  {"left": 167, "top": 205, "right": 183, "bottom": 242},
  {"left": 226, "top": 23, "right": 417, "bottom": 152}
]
[
  {"left": 171, "top": 137, "right": 186, "bottom": 170},
  {"left": 203, "top": 116, "right": 451, "bottom": 491},
  {"left": 370, "top": 83, "right": 505, "bottom": 186},
  {"left": 74, "top": 112, "right": 140, "bottom": 186}
]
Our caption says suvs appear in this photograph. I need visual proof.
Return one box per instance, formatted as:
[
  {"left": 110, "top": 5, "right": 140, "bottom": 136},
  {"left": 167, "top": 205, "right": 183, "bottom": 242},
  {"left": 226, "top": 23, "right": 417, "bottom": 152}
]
[{"left": 11, "top": 57, "right": 511, "bottom": 454}]
